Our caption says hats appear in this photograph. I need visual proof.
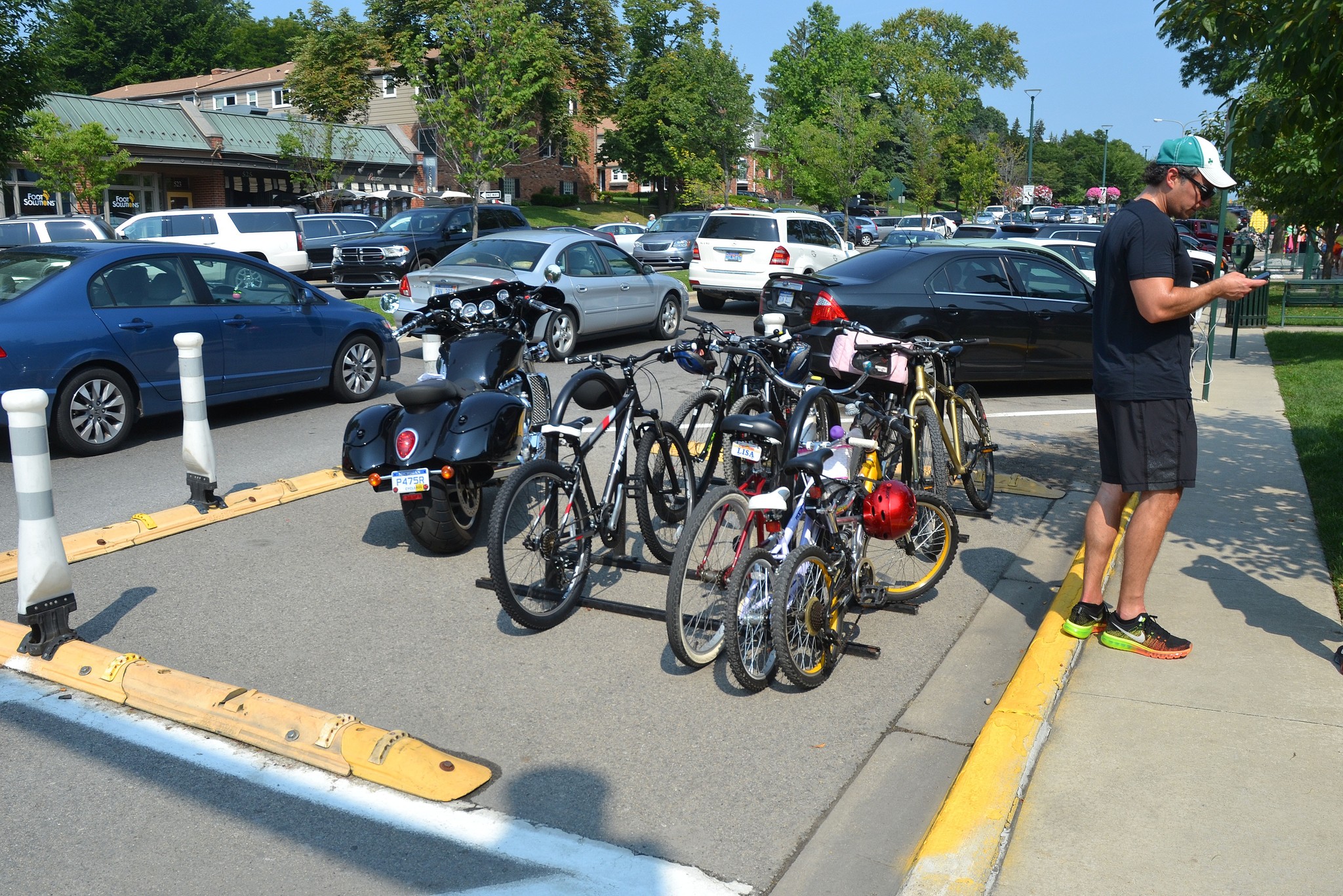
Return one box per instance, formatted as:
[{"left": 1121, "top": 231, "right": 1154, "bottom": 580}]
[{"left": 1156, "top": 134, "right": 1237, "bottom": 191}]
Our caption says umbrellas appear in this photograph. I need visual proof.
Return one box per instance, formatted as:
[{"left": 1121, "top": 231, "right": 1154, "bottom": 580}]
[
  {"left": 297, "top": 188, "right": 375, "bottom": 214},
  {"left": 412, "top": 188, "right": 472, "bottom": 205},
  {"left": 364, "top": 189, "right": 426, "bottom": 218}
]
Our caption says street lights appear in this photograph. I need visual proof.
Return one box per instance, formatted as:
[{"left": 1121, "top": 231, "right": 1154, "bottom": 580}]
[
  {"left": 1153, "top": 118, "right": 1219, "bottom": 138},
  {"left": 838, "top": 93, "right": 882, "bottom": 138},
  {"left": 1024, "top": 88, "right": 1043, "bottom": 185},
  {"left": 1101, "top": 124, "right": 1113, "bottom": 226},
  {"left": 1142, "top": 146, "right": 1150, "bottom": 160}
]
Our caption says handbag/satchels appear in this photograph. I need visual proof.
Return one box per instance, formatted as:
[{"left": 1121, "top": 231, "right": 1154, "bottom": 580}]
[{"left": 1333, "top": 243, "right": 1342, "bottom": 255}]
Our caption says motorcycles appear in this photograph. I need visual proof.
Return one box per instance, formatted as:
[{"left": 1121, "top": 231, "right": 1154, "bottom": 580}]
[{"left": 337, "top": 262, "right": 582, "bottom": 554}]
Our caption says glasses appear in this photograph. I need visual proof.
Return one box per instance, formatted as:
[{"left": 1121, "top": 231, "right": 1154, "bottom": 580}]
[{"left": 1177, "top": 171, "right": 1216, "bottom": 201}]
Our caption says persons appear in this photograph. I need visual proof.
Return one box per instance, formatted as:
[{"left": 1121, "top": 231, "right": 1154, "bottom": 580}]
[
  {"left": 1285, "top": 219, "right": 1343, "bottom": 277},
  {"left": 1060, "top": 132, "right": 1269, "bottom": 660},
  {"left": 448, "top": 217, "right": 467, "bottom": 233},
  {"left": 647, "top": 214, "right": 660, "bottom": 232},
  {"left": 623, "top": 216, "right": 633, "bottom": 233},
  {"left": 546, "top": 273, "right": 554, "bottom": 283}
]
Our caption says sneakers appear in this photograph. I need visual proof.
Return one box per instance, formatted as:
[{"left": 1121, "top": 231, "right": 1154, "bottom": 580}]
[
  {"left": 1062, "top": 604, "right": 1110, "bottom": 639},
  {"left": 1102, "top": 611, "right": 1193, "bottom": 659}
]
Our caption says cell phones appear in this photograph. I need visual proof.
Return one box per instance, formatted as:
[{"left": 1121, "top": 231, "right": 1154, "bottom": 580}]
[{"left": 1250, "top": 271, "right": 1271, "bottom": 280}]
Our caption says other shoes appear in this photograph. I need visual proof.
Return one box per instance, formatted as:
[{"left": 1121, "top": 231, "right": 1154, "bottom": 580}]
[
  {"left": 1340, "top": 273, "right": 1343, "bottom": 276},
  {"left": 1335, "top": 272, "right": 1338, "bottom": 275}
]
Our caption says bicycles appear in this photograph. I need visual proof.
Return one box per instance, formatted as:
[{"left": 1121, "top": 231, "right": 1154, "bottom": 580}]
[{"left": 484, "top": 313, "right": 1002, "bottom": 695}]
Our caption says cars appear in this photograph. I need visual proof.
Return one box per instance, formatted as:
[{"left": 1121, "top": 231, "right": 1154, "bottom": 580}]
[
  {"left": 1, "top": 244, "right": 403, "bottom": 456},
  {"left": 759, "top": 247, "right": 1099, "bottom": 404},
  {"left": 393, "top": 230, "right": 691, "bottom": 360},
  {"left": 773, "top": 200, "right": 1250, "bottom": 294},
  {"left": 592, "top": 223, "right": 653, "bottom": 258}
]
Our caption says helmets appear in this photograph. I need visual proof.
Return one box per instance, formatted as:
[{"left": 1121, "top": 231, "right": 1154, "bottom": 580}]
[
  {"left": 862, "top": 479, "right": 917, "bottom": 541},
  {"left": 776, "top": 336, "right": 812, "bottom": 384},
  {"left": 674, "top": 338, "right": 718, "bottom": 376}
]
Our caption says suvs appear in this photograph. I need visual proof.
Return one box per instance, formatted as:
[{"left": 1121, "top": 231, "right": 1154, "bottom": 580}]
[
  {"left": 634, "top": 213, "right": 707, "bottom": 267},
  {"left": 293, "top": 213, "right": 392, "bottom": 299},
  {"left": 691, "top": 205, "right": 863, "bottom": 311},
  {"left": 330, "top": 204, "right": 533, "bottom": 299},
  {"left": 113, "top": 205, "right": 309, "bottom": 283},
  {"left": 16, "top": 214, "right": 123, "bottom": 244}
]
